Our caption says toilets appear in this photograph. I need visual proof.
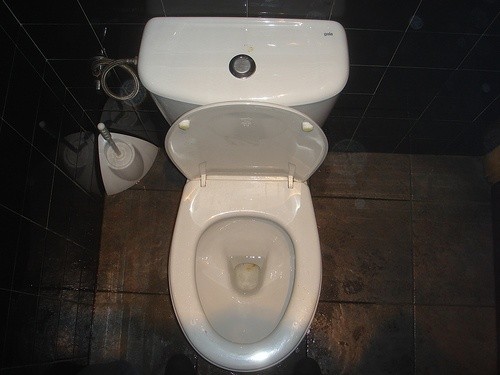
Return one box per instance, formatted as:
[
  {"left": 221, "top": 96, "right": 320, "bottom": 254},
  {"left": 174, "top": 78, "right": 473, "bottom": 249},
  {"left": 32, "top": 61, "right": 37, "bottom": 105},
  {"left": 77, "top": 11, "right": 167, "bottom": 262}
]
[{"left": 137, "top": 17, "right": 350, "bottom": 373}]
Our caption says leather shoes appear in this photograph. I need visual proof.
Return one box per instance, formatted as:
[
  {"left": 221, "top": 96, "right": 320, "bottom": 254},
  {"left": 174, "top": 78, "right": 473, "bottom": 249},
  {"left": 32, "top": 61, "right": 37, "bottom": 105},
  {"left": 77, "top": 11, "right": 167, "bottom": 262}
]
[
  {"left": 294, "top": 358, "right": 321, "bottom": 373},
  {"left": 164, "top": 354, "right": 194, "bottom": 375}
]
